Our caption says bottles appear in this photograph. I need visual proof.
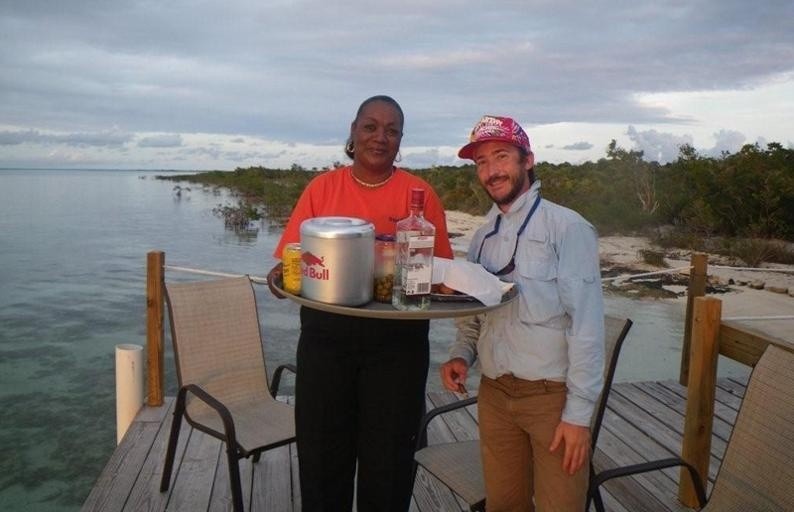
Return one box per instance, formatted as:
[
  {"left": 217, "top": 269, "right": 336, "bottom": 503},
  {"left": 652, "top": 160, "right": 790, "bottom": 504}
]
[{"left": 391, "top": 188, "right": 436, "bottom": 311}]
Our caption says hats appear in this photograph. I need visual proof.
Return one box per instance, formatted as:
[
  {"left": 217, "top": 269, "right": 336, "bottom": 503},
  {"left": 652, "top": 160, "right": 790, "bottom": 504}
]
[{"left": 457, "top": 115, "right": 530, "bottom": 160}]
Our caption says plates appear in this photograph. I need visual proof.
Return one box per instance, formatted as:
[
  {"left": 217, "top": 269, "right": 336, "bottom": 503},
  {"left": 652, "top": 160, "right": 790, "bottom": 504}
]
[{"left": 430, "top": 291, "right": 476, "bottom": 302}]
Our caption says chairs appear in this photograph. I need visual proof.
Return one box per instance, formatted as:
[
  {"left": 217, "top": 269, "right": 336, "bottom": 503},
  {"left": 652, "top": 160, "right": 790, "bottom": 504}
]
[
  {"left": 590, "top": 344, "right": 793, "bottom": 512},
  {"left": 401, "top": 309, "right": 634, "bottom": 512},
  {"left": 401, "top": 309, "right": 792, "bottom": 512},
  {"left": 155, "top": 276, "right": 303, "bottom": 510}
]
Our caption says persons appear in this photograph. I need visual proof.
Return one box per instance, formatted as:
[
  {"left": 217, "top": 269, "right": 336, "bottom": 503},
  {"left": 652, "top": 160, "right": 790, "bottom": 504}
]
[
  {"left": 437, "top": 114, "right": 608, "bottom": 512},
  {"left": 266, "top": 94, "right": 457, "bottom": 511}
]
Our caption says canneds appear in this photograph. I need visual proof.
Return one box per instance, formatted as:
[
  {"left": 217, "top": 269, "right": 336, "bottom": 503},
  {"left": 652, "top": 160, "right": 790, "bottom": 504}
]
[{"left": 282, "top": 242, "right": 303, "bottom": 295}]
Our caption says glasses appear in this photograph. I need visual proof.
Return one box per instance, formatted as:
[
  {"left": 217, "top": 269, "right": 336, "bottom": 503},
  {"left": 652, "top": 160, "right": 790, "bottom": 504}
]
[{"left": 476, "top": 233, "right": 520, "bottom": 276}]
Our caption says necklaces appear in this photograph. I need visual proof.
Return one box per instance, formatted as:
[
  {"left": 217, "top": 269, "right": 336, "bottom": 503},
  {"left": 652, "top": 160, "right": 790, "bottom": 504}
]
[{"left": 349, "top": 164, "right": 396, "bottom": 188}]
